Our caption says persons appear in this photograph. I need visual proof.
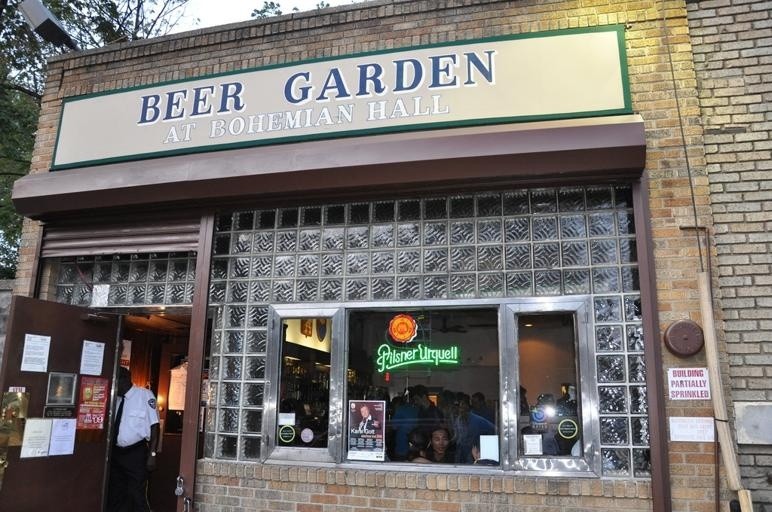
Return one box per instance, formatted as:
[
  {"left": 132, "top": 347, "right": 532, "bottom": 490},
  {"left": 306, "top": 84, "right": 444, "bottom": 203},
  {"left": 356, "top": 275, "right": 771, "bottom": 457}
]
[
  {"left": 275, "top": 378, "right": 581, "bottom": 465},
  {"left": 108, "top": 367, "right": 161, "bottom": 512}
]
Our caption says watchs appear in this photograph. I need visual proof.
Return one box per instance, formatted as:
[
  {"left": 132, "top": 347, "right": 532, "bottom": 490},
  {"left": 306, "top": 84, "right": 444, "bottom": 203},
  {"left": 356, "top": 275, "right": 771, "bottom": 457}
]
[{"left": 149, "top": 452, "right": 159, "bottom": 457}]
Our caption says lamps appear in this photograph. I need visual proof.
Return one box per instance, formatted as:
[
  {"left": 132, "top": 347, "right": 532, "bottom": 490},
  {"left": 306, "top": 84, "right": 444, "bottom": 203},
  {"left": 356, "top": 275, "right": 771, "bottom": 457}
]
[{"left": 17, "top": 0, "right": 80, "bottom": 52}]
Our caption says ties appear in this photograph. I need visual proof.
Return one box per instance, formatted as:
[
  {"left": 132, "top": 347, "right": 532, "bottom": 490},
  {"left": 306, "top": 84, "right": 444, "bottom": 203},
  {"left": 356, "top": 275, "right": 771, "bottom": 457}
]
[{"left": 111, "top": 395, "right": 127, "bottom": 448}]
